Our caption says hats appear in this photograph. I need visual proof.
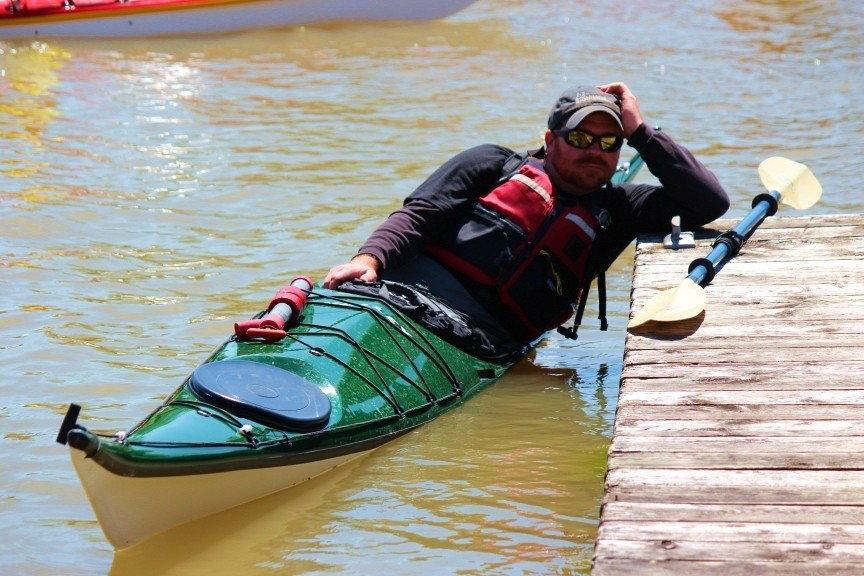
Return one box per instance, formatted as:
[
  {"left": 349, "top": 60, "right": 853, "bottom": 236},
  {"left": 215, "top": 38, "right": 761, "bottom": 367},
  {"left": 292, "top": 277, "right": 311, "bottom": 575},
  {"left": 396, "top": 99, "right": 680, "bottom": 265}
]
[{"left": 549, "top": 85, "right": 624, "bottom": 134}]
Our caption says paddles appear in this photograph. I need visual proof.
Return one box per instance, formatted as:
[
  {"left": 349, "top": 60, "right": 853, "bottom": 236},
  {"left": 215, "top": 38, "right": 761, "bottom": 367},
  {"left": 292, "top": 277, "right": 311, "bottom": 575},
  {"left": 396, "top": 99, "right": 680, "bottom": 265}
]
[{"left": 625, "top": 156, "right": 823, "bottom": 330}]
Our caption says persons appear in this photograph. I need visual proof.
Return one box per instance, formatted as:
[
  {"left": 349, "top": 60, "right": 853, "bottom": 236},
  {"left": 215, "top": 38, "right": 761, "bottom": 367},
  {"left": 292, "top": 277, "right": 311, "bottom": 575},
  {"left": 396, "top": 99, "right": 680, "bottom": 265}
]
[{"left": 323, "top": 84, "right": 729, "bottom": 364}]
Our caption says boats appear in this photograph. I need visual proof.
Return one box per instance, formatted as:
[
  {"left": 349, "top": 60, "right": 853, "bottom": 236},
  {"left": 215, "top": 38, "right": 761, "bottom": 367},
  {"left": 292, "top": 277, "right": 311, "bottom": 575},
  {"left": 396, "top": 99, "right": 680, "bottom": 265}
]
[
  {"left": 0, "top": 0, "right": 478, "bottom": 38},
  {"left": 53, "top": 124, "right": 664, "bottom": 552}
]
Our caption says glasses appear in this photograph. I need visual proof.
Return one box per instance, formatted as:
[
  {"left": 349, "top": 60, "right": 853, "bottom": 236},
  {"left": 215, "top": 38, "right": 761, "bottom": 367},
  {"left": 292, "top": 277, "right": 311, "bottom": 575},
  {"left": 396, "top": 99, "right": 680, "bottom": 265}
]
[{"left": 553, "top": 130, "right": 623, "bottom": 152}]
